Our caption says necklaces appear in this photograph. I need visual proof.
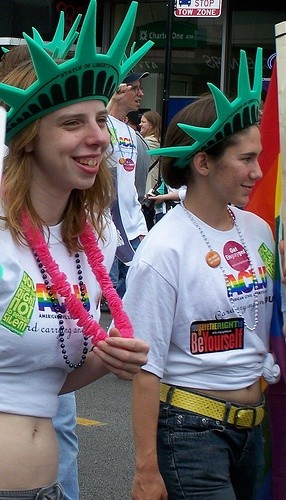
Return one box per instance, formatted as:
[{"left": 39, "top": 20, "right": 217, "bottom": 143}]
[
  {"left": 179, "top": 199, "right": 259, "bottom": 331},
  {"left": 31, "top": 239, "right": 88, "bottom": 369},
  {"left": 108, "top": 114, "right": 133, "bottom": 165}
]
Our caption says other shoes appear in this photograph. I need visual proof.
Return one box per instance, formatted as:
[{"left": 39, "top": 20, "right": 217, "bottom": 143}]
[{"left": 100, "top": 301, "right": 109, "bottom": 312}]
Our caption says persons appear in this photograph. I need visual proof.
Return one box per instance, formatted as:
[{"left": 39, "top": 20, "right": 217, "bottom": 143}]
[
  {"left": 100, "top": 66, "right": 187, "bottom": 313},
  {"left": 0, "top": 45, "right": 80, "bottom": 500},
  {"left": 0, "top": 58, "right": 150, "bottom": 500},
  {"left": 107, "top": 94, "right": 286, "bottom": 500}
]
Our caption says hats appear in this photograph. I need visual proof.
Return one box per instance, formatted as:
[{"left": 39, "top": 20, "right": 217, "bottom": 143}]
[{"left": 122, "top": 66, "right": 150, "bottom": 85}]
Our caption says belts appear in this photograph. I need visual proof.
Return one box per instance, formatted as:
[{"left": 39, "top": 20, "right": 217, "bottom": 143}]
[{"left": 159, "top": 382, "right": 267, "bottom": 428}]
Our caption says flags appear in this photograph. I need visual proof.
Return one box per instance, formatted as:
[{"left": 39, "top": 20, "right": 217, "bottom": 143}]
[{"left": 243, "top": 58, "right": 286, "bottom": 500}]
